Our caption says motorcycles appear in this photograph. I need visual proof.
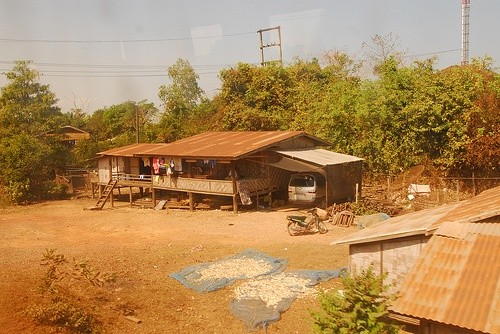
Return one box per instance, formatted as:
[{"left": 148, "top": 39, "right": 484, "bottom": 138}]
[{"left": 286, "top": 208, "right": 327, "bottom": 236}]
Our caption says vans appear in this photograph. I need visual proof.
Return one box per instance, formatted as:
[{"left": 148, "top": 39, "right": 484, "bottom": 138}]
[{"left": 288, "top": 173, "right": 326, "bottom": 207}]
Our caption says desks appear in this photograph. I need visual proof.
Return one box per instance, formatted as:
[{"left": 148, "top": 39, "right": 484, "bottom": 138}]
[{"left": 194, "top": 174, "right": 214, "bottom": 179}]
